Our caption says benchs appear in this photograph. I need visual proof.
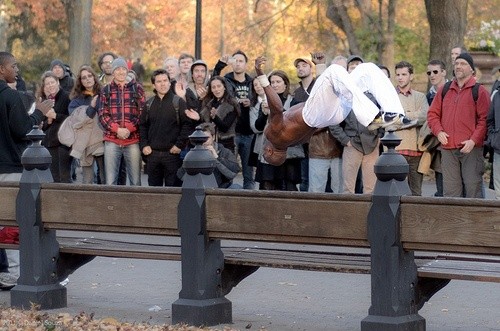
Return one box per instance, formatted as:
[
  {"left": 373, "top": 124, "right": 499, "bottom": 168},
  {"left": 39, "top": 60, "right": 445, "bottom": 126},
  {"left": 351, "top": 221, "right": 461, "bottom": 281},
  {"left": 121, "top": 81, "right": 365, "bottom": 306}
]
[{"left": 0, "top": 126, "right": 500, "bottom": 331}]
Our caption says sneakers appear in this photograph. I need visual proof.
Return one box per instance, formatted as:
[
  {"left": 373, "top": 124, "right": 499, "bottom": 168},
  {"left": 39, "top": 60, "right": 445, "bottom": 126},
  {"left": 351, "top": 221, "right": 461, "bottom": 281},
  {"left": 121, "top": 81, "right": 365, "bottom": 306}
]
[
  {"left": 367, "top": 112, "right": 402, "bottom": 132},
  {"left": 385, "top": 113, "right": 419, "bottom": 133}
]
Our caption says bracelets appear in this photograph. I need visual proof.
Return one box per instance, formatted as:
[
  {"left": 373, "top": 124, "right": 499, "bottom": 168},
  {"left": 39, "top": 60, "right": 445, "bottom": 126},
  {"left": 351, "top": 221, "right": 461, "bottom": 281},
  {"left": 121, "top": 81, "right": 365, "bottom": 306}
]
[{"left": 257, "top": 74, "right": 270, "bottom": 88}]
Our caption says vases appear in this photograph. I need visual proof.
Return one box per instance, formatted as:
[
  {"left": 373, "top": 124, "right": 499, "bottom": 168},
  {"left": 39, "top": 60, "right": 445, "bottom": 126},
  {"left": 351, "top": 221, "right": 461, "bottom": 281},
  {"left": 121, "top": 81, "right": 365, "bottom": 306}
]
[{"left": 468, "top": 51, "right": 500, "bottom": 85}]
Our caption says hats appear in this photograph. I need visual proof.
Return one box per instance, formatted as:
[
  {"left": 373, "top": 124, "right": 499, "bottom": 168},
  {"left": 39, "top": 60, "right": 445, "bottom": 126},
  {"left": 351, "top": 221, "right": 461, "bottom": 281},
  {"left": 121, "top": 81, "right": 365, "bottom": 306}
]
[
  {"left": 98, "top": 52, "right": 118, "bottom": 68},
  {"left": 346, "top": 55, "right": 364, "bottom": 63},
  {"left": 294, "top": 57, "right": 312, "bottom": 68},
  {"left": 110, "top": 57, "right": 128, "bottom": 72},
  {"left": 455, "top": 53, "right": 475, "bottom": 71},
  {"left": 51, "top": 60, "right": 66, "bottom": 74}
]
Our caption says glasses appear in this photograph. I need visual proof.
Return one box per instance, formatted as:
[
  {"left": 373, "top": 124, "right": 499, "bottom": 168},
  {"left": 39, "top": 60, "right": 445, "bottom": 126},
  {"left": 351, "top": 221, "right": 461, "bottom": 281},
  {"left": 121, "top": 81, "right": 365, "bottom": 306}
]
[
  {"left": 427, "top": 70, "right": 438, "bottom": 76},
  {"left": 103, "top": 61, "right": 112, "bottom": 65}
]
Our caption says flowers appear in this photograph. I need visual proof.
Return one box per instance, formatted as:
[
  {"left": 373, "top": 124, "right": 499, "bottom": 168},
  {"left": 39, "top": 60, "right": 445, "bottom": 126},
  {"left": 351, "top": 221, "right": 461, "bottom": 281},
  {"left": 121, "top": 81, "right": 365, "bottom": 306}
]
[{"left": 464, "top": 18, "right": 500, "bottom": 57}]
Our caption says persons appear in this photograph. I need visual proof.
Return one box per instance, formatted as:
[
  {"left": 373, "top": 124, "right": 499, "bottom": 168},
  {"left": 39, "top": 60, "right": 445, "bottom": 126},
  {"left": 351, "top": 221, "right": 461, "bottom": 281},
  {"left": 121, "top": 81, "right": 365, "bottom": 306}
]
[{"left": 0, "top": 50, "right": 500, "bottom": 198}]
